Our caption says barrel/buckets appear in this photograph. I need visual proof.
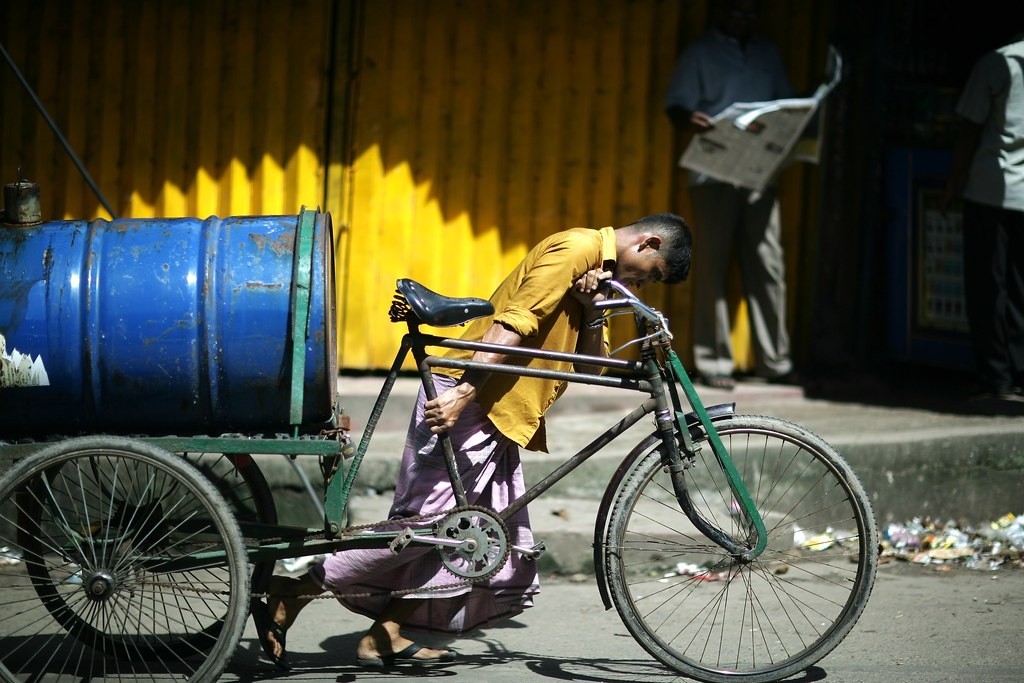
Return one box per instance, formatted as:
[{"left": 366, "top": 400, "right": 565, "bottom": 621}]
[{"left": 0, "top": 205, "right": 342, "bottom": 445}]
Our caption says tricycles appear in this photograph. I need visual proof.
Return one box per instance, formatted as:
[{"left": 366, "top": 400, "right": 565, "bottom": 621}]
[{"left": 0, "top": 281, "right": 880, "bottom": 683}]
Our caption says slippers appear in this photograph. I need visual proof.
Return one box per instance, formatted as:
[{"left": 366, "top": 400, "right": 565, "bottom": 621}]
[
  {"left": 357, "top": 641, "right": 458, "bottom": 667},
  {"left": 250, "top": 599, "right": 290, "bottom": 672}
]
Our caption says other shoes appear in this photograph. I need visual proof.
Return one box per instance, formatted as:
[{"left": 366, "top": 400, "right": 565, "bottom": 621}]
[
  {"left": 704, "top": 374, "right": 733, "bottom": 389},
  {"left": 767, "top": 371, "right": 801, "bottom": 388}
]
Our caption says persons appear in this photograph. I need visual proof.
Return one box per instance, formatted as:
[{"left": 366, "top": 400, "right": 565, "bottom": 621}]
[
  {"left": 935, "top": 38, "right": 1024, "bottom": 411},
  {"left": 669, "top": 0, "right": 798, "bottom": 389},
  {"left": 250, "top": 214, "right": 692, "bottom": 668}
]
